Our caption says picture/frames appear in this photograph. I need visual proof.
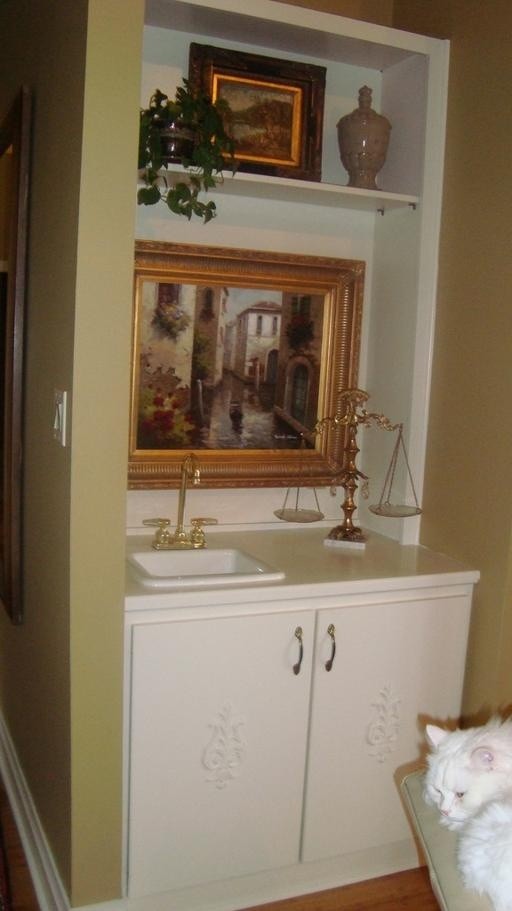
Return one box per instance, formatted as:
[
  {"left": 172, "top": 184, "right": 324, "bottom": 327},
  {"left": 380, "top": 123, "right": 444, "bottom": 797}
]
[
  {"left": 130, "top": 238, "right": 366, "bottom": 488},
  {"left": 190, "top": 43, "right": 327, "bottom": 182}
]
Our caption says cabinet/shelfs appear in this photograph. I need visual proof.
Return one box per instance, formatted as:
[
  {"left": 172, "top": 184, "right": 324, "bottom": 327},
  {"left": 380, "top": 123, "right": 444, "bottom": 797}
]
[
  {"left": 125, "top": 583, "right": 473, "bottom": 909},
  {"left": 129, "top": 0, "right": 450, "bottom": 549}
]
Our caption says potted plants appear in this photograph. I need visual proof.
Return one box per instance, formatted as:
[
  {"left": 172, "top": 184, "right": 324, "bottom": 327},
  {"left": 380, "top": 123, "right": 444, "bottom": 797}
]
[{"left": 136, "top": 76, "right": 242, "bottom": 223}]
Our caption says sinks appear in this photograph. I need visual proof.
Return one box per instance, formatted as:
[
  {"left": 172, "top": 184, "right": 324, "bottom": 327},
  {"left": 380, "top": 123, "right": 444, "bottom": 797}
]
[{"left": 126, "top": 547, "right": 285, "bottom": 587}]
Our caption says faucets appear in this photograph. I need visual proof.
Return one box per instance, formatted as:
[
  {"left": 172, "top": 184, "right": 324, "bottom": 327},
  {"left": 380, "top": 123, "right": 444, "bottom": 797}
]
[{"left": 177, "top": 453, "right": 200, "bottom": 530}]
